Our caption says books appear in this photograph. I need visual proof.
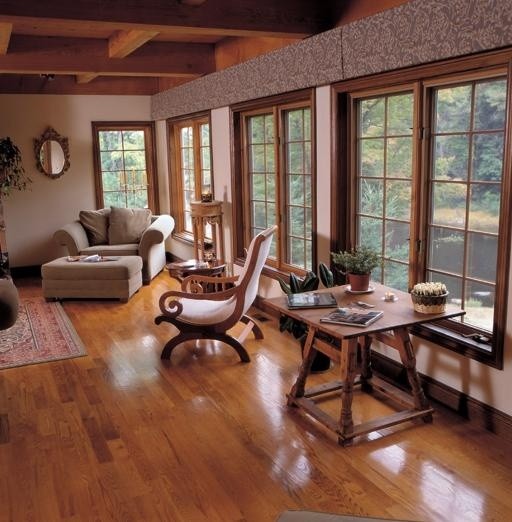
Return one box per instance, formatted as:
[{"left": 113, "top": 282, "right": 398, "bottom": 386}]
[
  {"left": 287, "top": 292, "right": 338, "bottom": 309},
  {"left": 320, "top": 307, "right": 383, "bottom": 327}
]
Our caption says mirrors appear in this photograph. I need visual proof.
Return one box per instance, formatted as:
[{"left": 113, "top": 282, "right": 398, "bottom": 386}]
[{"left": 32, "top": 127, "right": 71, "bottom": 181}]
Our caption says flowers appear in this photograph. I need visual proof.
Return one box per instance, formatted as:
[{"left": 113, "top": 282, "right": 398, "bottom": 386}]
[{"left": 410, "top": 280, "right": 447, "bottom": 296}]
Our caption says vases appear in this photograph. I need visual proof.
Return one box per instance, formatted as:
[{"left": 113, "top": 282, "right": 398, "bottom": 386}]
[{"left": 407, "top": 289, "right": 452, "bottom": 319}]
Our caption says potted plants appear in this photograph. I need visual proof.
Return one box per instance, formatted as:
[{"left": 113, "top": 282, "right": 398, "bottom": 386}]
[
  {"left": 328, "top": 243, "right": 388, "bottom": 292},
  {"left": 273, "top": 258, "right": 338, "bottom": 372}
]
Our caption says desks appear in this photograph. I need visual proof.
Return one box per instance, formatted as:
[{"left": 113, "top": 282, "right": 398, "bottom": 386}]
[
  {"left": 187, "top": 198, "right": 227, "bottom": 292},
  {"left": 258, "top": 275, "right": 467, "bottom": 450},
  {"left": 164, "top": 256, "right": 227, "bottom": 291}
]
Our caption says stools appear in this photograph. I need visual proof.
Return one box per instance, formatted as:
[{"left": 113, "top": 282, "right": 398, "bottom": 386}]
[
  {"left": 39, "top": 252, "right": 143, "bottom": 304},
  {"left": 0, "top": 277, "right": 23, "bottom": 329}
]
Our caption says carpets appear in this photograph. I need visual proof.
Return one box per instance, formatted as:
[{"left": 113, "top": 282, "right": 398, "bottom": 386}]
[{"left": 1, "top": 294, "right": 91, "bottom": 372}]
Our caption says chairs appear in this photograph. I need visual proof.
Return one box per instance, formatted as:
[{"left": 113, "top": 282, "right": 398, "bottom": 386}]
[
  {"left": 51, "top": 212, "right": 178, "bottom": 288},
  {"left": 150, "top": 223, "right": 279, "bottom": 367}
]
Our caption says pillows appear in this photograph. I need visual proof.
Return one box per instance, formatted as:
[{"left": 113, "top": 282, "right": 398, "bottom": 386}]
[
  {"left": 79, "top": 207, "right": 114, "bottom": 247},
  {"left": 105, "top": 205, "right": 153, "bottom": 246}
]
